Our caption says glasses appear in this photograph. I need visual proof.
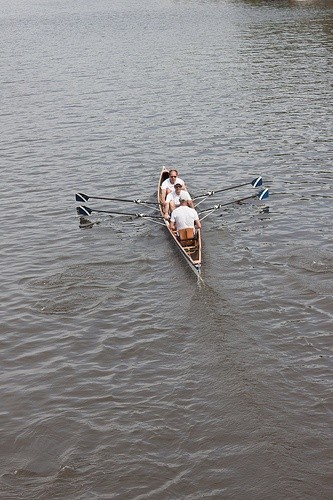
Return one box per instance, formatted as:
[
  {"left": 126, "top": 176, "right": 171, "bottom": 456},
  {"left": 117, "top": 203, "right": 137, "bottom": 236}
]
[
  {"left": 170, "top": 176, "right": 177, "bottom": 178},
  {"left": 176, "top": 186, "right": 182, "bottom": 188}
]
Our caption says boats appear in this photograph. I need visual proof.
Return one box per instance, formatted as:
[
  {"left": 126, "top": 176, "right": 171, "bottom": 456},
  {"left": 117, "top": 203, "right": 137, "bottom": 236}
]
[{"left": 159, "top": 167, "right": 204, "bottom": 279}]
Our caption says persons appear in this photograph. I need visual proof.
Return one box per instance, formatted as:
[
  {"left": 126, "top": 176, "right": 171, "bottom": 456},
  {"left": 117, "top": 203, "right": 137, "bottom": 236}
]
[
  {"left": 160, "top": 169, "right": 185, "bottom": 207},
  {"left": 168, "top": 193, "right": 202, "bottom": 244},
  {"left": 164, "top": 181, "right": 195, "bottom": 218}
]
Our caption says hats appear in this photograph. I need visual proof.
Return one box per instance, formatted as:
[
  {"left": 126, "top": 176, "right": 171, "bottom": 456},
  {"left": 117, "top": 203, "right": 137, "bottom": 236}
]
[
  {"left": 180, "top": 193, "right": 188, "bottom": 202},
  {"left": 174, "top": 181, "right": 184, "bottom": 186}
]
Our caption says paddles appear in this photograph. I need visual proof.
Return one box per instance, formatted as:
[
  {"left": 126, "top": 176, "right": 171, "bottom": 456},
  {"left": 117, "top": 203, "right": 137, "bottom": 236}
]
[
  {"left": 191, "top": 175, "right": 263, "bottom": 200},
  {"left": 197, "top": 187, "right": 270, "bottom": 215},
  {"left": 135, "top": 212, "right": 175, "bottom": 229},
  {"left": 76, "top": 205, "right": 171, "bottom": 220},
  {"left": 134, "top": 198, "right": 163, "bottom": 212},
  {"left": 195, "top": 191, "right": 215, "bottom": 209},
  {"left": 78, "top": 216, "right": 121, "bottom": 228},
  {"left": 199, "top": 203, "right": 222, "bottom": 222},
  {"left": 75, "top": 193, "right": 161, "bottom": 205}
]
